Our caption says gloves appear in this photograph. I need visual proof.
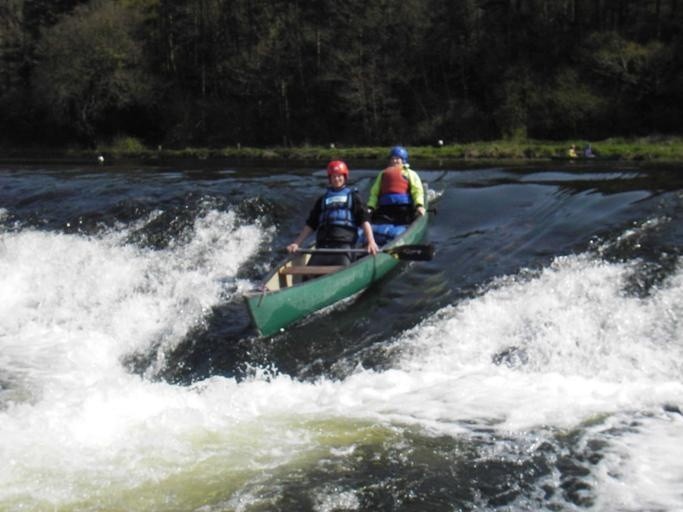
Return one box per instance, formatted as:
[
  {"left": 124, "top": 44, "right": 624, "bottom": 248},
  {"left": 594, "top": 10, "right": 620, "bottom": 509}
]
[
  {"left": 551, "top": 155, "right": 621, "bottom": 161},
  {"left": 244, "top": 183, "right": 431, "bottom": 335}
]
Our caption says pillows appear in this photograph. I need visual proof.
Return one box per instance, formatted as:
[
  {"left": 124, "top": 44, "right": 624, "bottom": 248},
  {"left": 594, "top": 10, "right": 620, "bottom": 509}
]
[
  {"left": 327, "top": 160, "right": 349, "bottom": 177},
  {"left": 390, "top": 145, "right": 408, "bottom": 161}
]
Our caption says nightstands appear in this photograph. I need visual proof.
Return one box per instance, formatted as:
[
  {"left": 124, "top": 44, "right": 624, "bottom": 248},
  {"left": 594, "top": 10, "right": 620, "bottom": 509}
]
[{"left": 257, "top": 245, "right": 435, "bottom": 260}]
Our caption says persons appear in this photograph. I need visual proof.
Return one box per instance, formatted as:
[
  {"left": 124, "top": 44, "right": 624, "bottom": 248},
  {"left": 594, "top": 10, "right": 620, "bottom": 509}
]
[
  {"left": 284, "top": 160, "right": 380, "bottom": 283},
  {"left": 355, "top": 145, "right": 426, "bottom": 250}
]
